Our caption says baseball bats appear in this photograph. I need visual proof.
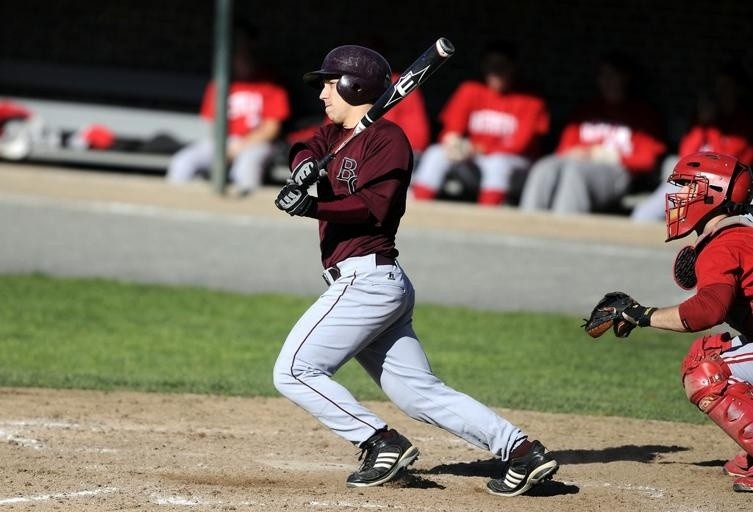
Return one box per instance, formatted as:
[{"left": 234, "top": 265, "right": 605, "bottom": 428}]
[{"left": 314, "top": 37, "right": 455, "bottom": 170}]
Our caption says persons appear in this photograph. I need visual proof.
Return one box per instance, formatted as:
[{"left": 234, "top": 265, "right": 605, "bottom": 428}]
[
  {"left": 405, "top": 48, "right": 555, "bottom": 209},
  {"left": 284, "top": 61, "right": 430, "bottom": 175},
  {"left": 269, "top": 43, "right": 562, "bottom": 499},
  {"left": 631, "top": 80, "right": 753, "bottom": 221},
  {"left": 511, "top": 54, "right": 665, "bottom": 215},
  {"left": 164, "top": 44, "right": 292, "bottom": 194},
  {"left": 577, "top": 125, "right": 753, "bottom": 498}
]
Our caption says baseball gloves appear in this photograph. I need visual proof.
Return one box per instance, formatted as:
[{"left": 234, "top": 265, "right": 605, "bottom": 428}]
[{"left": 580, "top": 291, "right": 639, "bottom": 337}]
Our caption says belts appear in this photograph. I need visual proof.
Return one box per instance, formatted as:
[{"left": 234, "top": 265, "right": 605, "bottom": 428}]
[{"left": 323, "top": 254, "right": 395, "bottom": 285}]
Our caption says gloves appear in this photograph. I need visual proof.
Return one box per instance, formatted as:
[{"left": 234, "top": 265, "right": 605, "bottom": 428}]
[
  {"left": 291, "top": 157, "right": 329, "bottom": 192},
  {"left": 277, "top": 180, "right": 317, "bottom": 218}
]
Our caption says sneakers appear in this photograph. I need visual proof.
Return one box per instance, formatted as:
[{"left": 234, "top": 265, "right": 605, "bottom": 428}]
[
  {"left": 484, "top": 441, "right": 559, "bottom": 496},
  {"left": 346, "top": 428, "right": 419, "bottom": 487},
  {"left": 722, "top": 453, "right": 752, "bottom": 478},
  {"left": 732, "top": 476, "right": 752, "bottom": 492}
]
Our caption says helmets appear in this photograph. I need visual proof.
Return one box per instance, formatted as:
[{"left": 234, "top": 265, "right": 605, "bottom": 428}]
[
  {"left": 663, "top": 151, "right": 753, "bottom": 243},
  {"left": 302, "top": 46, "right": 393, "bottom": 106}
]
